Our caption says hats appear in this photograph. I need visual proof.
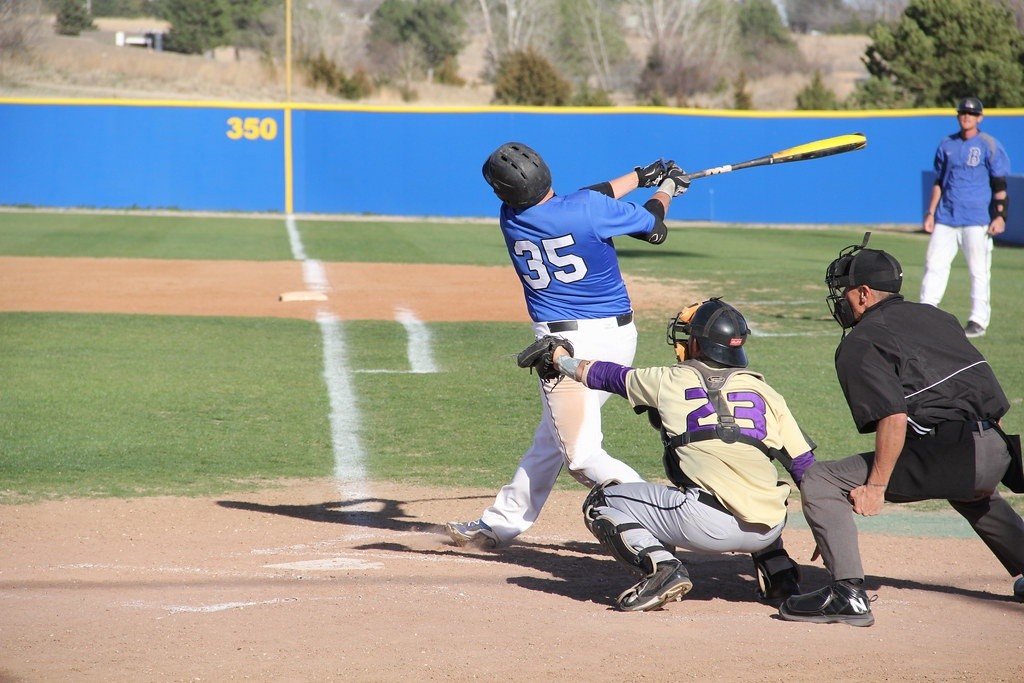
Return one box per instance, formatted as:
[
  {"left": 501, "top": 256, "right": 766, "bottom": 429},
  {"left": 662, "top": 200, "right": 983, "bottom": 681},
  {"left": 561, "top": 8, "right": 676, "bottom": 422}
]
[{"left": 957, "top": 97, "right": 983, "bottom": 115}]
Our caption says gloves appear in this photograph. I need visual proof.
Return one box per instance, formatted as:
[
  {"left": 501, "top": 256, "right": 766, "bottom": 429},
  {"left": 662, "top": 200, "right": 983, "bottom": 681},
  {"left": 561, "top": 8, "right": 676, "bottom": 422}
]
[
  {"left": 663, "top": 163, "right": 691, "bottom": 197},
  {"left": 635, "top": 160, "right": 674, "bottom": 188}
]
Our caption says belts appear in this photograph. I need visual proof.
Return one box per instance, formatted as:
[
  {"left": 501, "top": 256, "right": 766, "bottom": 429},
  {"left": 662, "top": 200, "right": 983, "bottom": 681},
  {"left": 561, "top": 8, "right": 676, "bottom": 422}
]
[
  {"left": 934, "top": 419, "right": 993, "bottom": 432},
  {"left": 677, "top": 483, "right": 734, "bottom": 516},
  {"left": 548, "top": 311, "right": 633, "bottom": 333}
]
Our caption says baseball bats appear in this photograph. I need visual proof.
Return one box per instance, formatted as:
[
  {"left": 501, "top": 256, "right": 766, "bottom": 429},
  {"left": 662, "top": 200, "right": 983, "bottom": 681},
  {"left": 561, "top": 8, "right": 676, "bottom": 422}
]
[{"left": 687, "top": 132, "right": 867, "bottom": 179}]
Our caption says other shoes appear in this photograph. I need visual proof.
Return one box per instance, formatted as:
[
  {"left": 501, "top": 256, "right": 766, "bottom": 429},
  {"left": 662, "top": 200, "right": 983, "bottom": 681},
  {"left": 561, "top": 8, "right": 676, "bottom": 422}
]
[
  {"left": 779, "top": 582, "right": 878, "bottom": 627},
  {"left": 964, "top": 320, "right": 985, "bottom": 337}
]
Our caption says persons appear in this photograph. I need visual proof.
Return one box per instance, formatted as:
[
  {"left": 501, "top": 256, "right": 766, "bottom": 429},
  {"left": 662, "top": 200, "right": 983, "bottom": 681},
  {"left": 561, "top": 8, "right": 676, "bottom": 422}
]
[
  {"left": 515, "top": 297, "right": 818, "bottom": 613},
  {"left": 443, "top": 142, "right": 689, "bottom": 561},
  {"left": 777, "top": 243, "right": 1024, "bottom": 627},
  {"left": 920, "top": 96, "right": 1011, "bottom": 337}
]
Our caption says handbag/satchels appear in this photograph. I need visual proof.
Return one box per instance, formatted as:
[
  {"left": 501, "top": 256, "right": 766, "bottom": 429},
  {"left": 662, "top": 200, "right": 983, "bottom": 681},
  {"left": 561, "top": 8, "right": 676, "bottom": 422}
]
[
  {"left": 887, "top": 422, "right": 975, "bottom": 500},
  {"left": 998, "top": 432, "right": 1024, "bottom": 494}
]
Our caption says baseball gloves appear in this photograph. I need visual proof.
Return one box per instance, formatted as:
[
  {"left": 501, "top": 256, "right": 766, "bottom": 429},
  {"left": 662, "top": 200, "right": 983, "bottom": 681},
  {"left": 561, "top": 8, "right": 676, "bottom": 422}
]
[{"left": 517, "top": 335, "right": 574, "bottom": 380}]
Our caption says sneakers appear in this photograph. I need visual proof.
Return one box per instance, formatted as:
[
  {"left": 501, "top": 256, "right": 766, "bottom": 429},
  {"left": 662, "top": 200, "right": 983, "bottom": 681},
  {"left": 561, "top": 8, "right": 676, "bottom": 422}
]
[
  {"left": 751, "top": 537, "right": 801, "bottom": 606},
  {"left": 446, "top": 519, "right": 501, "bottom": 549},
  {"left": 620, "top": 559, "right": 693, "bottom": 611}
]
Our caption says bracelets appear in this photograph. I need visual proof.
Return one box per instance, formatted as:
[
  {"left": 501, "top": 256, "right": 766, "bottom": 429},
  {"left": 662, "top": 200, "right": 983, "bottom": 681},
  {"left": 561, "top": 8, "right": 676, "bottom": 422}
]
[{"left": 927, "top": 212, "right": 934, "bottom": 216}]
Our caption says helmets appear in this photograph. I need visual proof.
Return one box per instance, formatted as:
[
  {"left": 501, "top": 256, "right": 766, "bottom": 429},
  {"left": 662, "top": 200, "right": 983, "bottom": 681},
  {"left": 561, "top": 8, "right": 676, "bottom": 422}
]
[
  {"left": 685, "top": 301, "right": 748, "bottom": 368},
  {"left": 481, "top": 142, "right": 552, "bottom": 209},
  {"left": 831, "top": 249, "right": 903, "bottom": 293}
]
